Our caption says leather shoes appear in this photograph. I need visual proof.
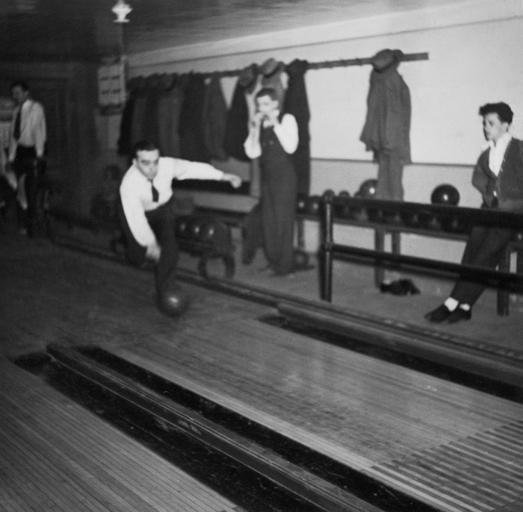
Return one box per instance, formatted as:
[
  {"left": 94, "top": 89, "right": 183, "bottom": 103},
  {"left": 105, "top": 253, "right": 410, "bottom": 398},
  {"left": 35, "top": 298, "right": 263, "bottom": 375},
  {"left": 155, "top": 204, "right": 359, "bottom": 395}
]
[
  {"left": 380, "top": 279, "right": 420, "bottom": 295},
  {"left": 425, "top": 304, "right": 471, "bottom": 324}
]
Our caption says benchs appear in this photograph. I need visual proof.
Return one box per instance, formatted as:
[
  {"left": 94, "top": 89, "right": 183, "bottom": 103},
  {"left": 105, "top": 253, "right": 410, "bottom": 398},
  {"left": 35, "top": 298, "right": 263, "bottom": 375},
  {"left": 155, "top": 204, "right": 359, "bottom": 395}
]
[
  {"left": 295, "top": 193, "right": 523, "bottom": 318},
  {"left": 29, "top": 181, "right": 257, "bottom": 281}
]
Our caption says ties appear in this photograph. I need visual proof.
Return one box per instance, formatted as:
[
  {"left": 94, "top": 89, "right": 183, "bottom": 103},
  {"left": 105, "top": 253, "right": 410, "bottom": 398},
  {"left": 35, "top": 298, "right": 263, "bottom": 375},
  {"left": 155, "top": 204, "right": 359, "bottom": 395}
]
[
  {"left": 14, "top": 104, "right": 22, "bottom": 142},
  {"left": 152, "top": 186, "right": 158, "bottom": 202}
]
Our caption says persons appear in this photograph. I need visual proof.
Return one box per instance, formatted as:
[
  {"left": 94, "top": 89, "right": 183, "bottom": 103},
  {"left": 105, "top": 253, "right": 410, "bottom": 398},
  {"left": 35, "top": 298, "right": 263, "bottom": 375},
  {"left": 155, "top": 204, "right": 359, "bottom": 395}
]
[
  {"left": 9, "top": 82, "right": 48, "bottom": 230},
  {"left": 120, "top": 140, "right": 241, "bottom": 295},
  {"left": 427, "top": 102, "right": 523, "bottom": 324},
  {"left": 245, "top": 89, "right": 300, "bottom": 278}
]
